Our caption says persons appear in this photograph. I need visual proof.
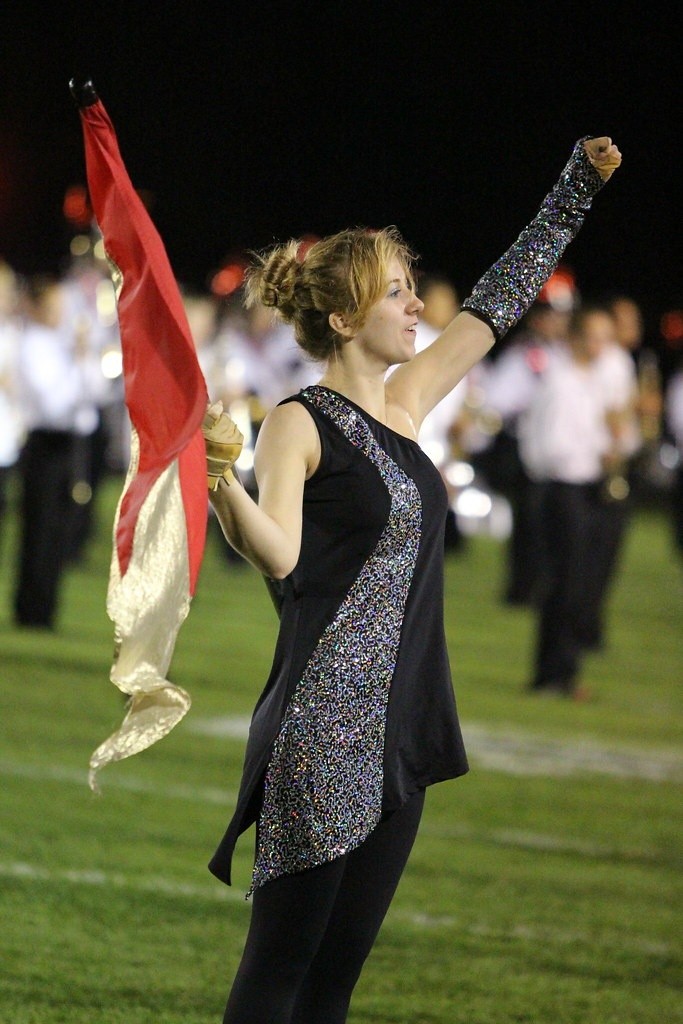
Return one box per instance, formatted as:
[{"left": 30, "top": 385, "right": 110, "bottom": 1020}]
[
  {"left": 0, "top": 243, "right": 682, "bottom": 694},
  {"left": 200, "top": 136, "right": 622, "bottom": 1022}
]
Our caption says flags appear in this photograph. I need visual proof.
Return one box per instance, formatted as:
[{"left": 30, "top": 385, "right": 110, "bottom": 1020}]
[{"left": 81, "top": 98, "right": 211, "bottom": 788}]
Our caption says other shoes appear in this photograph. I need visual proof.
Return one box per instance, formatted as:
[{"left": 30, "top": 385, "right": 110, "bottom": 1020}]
[{"left": 532, "top": 680, "right": 593, "bottom": 706}]
